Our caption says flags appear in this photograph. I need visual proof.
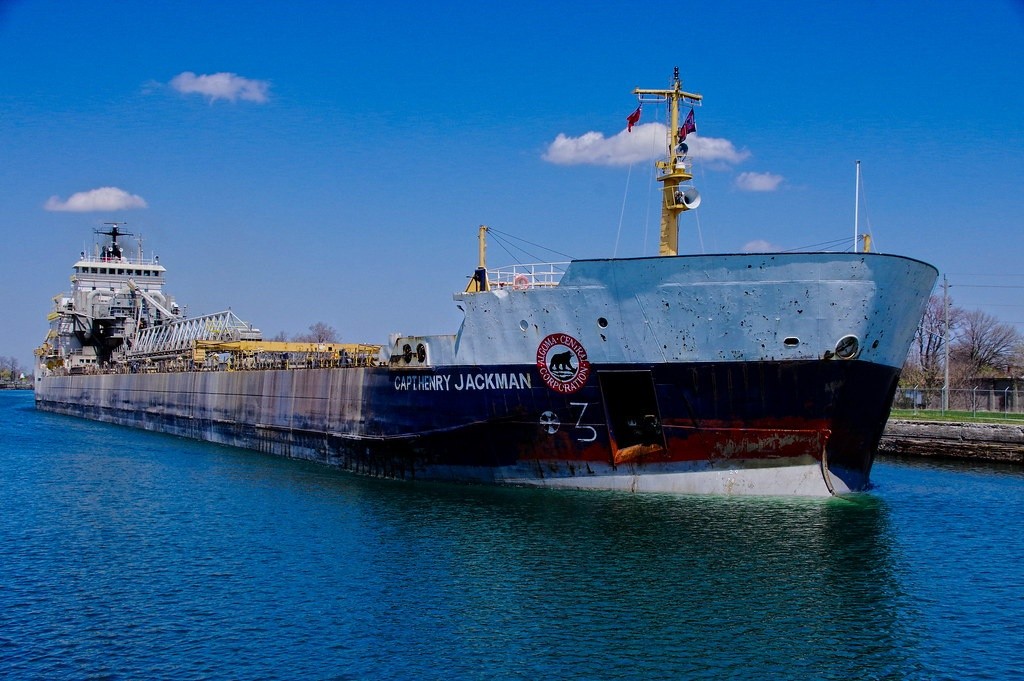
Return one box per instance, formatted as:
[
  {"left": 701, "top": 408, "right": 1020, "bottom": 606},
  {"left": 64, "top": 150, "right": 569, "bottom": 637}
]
[
  {"left": 627, "top": 104, "right": 641, "bottom": 132},
  {"left": 678, "top": 109, "right": 696, "bottom": 143}
]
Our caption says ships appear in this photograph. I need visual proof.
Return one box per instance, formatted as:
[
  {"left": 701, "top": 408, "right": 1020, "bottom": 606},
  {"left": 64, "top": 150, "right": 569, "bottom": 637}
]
[{"left": 33, "top": 65, "right": 941, "bottom": 513}]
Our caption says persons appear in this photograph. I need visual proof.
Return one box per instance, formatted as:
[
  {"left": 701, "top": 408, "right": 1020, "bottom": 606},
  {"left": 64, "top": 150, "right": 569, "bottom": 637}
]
[
  {"left": 40, "top": 315, "right": 370, "bottom": 374},
  {"left": 80, "top": 252, "right": 85, "bottom": 262},
  {"left": 100, "top": 245, "right": 111, "bottom": 260}
]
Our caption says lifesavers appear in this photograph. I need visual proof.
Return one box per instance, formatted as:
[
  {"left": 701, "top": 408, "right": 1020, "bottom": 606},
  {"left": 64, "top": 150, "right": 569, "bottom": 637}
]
[{"left": 515, "top": 274, "right": 529, "bottom": 290}]
